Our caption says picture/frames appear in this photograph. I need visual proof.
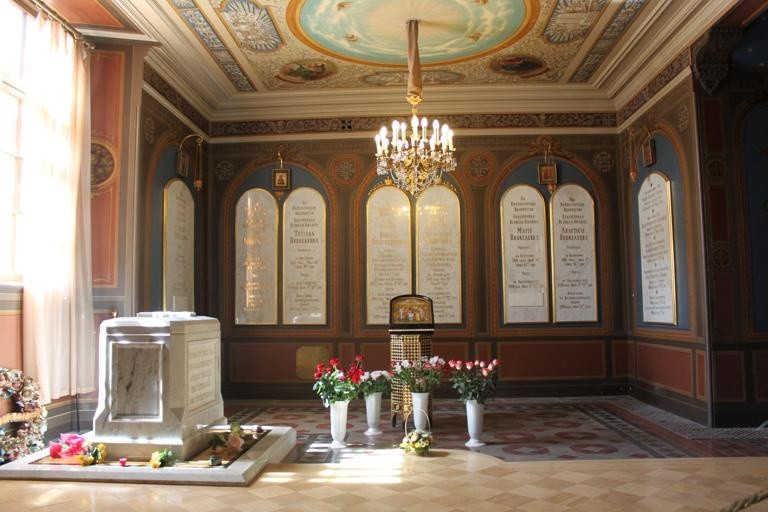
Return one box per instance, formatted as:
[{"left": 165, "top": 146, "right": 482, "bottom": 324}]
[
  {"left": 537, "top": 162, "right": 558, "bottom": 186},
  {"left": 271, "top": 169, "right": 291, "bottom": 192}
]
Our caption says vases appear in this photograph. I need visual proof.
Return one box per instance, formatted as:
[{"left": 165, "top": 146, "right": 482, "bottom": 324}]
[{"left": 326, "top": 391, "right": 487, "bottom": 448}]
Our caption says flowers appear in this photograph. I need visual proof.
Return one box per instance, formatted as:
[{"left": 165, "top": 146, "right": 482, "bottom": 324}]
[
  {"left": 49, "top": 431, "right": 107, "bottom": 465},
  {"left": 313, "top": 352, "right": 503, "bottom": 409},
  {"left": 399, "top": 428, "right": 433, "bottom": 452},
  {"left": 149, "top": 448, "right": 177, "bottom": 469}
]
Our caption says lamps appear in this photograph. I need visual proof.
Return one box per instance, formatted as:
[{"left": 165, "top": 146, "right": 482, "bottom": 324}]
[{"left": 373, "top": 20, "right": 456, "bottom": 199}]
